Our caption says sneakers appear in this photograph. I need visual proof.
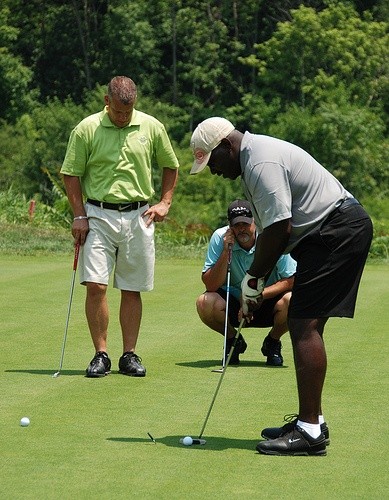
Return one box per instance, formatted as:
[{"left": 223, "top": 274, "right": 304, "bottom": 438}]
[
  {"left": 118, "top": 353, "right": 146, "bottom": 377},
  {"left": 261, "top": 338, "right": 283, "bottom": 366},
  {"left": 86, "top": 352, "right": 111, "bottom": 376},
  {"left": 257, "top": 421, "right": 327, "bottom": 456},
  {"left": 261, "top": 413, "right": 330, "bottom": 445},
  {"left": 221, "top": 332, "right": 247, "bottom": 365}
]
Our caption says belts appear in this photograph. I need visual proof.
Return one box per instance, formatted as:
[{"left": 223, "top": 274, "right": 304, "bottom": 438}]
[
  {"left": 87, "top": 198, "right": 148, "bottom": 212},
  {"left": 329, "top": 198, "right": 359, "bottom": 215}
]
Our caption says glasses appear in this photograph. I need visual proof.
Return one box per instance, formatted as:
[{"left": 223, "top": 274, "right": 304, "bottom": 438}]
[{"left": 229, "top": 209, "right": 252, "bottom": 219}]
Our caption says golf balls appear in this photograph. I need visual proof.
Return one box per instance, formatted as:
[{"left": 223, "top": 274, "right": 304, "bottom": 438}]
[
  {"left": 183, "top": 437, "right": 193, "bottom": 446},
  {"left": 20, "top": 417, "right": 30, "bottom": 427}
]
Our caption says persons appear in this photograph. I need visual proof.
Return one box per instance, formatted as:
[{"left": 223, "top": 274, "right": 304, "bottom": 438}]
[
  {"left": 196, "top": 199, "right": 297, "bottom": 365},
  {"left": 190, "top": 117, "right": 373, "bottom": 457},
  {"left": 60, "top": 77, "right": 180, "bottom": 376}
]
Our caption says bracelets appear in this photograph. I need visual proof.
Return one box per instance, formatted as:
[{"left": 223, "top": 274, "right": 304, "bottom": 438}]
[{"left": 74, "top": 216, "right": 88, "bottom": 220}]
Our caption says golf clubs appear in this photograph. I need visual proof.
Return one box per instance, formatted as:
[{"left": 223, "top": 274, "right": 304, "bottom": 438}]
[
  {"left": 211, "top": 243, "right": 233, "bottom": 373},
  {"left": 193, "top": 318, "right": 245, "bottom": 446},
  {"left": 52, "top": 244, "right": 80, "bottom": 378}
]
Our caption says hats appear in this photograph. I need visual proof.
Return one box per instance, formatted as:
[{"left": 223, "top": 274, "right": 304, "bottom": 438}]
[
  {"left": 228, "top": 198, "right": 254, "bottom": 226},
  {"left": 189, "top": 117, "right": 235, "bottom": 174}
]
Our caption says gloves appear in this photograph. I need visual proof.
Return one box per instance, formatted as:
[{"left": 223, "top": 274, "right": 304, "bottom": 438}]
[{"left": 241, "top": 270, "right": 265, "bottom": 316}]
